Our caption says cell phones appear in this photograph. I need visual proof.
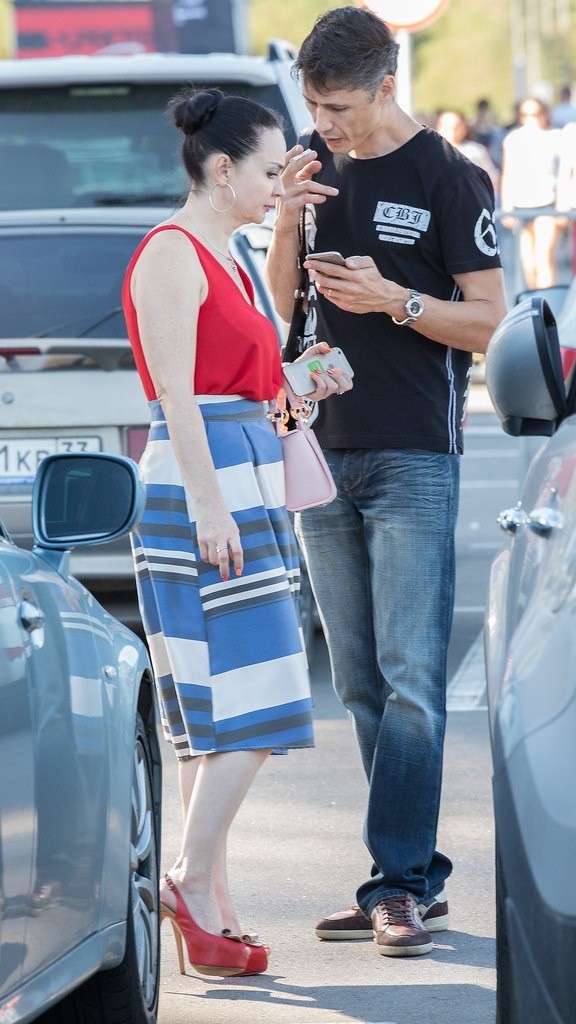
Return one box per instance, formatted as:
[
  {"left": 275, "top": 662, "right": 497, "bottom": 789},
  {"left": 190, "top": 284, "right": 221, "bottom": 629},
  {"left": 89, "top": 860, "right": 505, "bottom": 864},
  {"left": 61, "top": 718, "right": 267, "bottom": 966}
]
[
  {"left": 305, "top": 250, "right": 347, "bottom": 277},
  {"left": 282, "top": 347, "right": 355, "bottom": 396}
]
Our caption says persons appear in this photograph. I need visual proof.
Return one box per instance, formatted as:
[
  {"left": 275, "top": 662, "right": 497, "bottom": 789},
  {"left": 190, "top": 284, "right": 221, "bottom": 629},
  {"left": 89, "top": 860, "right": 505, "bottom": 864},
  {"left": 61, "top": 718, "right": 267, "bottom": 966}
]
[
  {"left": 266, "top": 6, "right": 506, "bottom": 958},
  {"left": 415, "top": 86, "right": 576, "bottom": 292},
  {"left": 129, "top": 89, "right": 353, "bottom": 978}
]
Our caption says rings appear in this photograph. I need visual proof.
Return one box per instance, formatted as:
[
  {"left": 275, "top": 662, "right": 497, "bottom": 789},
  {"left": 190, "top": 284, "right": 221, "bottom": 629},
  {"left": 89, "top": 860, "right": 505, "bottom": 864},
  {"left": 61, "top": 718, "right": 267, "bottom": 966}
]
[
  {"left": 216, "top": 545, "right": 228, "bottom": 553},
  {"left": 327, "top": 289, "right": 332, "bottom": 297},
  {"left": 336, "top": 390, "right": 345, "bottom": 395}
]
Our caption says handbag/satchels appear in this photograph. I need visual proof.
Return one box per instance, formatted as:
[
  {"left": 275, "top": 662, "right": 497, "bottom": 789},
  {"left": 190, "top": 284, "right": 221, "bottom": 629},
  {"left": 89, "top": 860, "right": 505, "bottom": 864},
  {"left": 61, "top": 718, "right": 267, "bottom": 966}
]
[{"left": 279, "top": 428, "right": 337, "bottom": 511}]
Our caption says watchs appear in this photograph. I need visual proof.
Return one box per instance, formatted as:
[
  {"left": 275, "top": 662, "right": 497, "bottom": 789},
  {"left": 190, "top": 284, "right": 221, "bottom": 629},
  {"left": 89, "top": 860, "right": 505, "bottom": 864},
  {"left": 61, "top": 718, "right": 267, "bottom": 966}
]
[{"left": 392, "top": 288, "right": 424, "bottom": 327}]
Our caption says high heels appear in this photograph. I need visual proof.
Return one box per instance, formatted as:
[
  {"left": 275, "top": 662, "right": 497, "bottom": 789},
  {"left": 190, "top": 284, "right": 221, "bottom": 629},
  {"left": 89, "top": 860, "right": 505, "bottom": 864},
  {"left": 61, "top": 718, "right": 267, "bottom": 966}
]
[
  {"left": 170, "top": 920, "right": 271, "bottom": 974},
  {"left": 159, "top": 873, "right": 252, "bottom": 975}
]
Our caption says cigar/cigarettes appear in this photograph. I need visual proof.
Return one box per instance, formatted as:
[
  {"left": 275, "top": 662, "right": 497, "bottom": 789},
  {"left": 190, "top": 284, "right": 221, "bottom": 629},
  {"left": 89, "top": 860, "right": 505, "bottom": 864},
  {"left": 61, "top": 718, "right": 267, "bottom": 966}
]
[{"left": 289, "top": 149, "right": 311, "bottom": 162}]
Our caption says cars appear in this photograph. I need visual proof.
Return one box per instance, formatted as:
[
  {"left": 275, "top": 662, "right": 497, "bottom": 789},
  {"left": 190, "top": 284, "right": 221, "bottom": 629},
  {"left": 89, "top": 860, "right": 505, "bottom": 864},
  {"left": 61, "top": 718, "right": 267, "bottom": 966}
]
[
  {"left": 466, "top": 253, "right": 576, "bottom": 1024},
  {"left": 0, "top": 207, "right": 186, "bottom": 604},
  {"left": 1, "top": 446, "right": 172, "bottom": 1024}
]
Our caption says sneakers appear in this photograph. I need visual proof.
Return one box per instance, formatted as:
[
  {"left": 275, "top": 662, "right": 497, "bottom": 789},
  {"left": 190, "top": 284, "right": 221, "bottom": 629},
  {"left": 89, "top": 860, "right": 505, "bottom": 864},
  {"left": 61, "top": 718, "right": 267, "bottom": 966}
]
[
  {"left": 315, "top": 886, "right": 449, "bottom": 939},
  {"left": 371, "top": 895, "right": 433, "bottom": 956}
]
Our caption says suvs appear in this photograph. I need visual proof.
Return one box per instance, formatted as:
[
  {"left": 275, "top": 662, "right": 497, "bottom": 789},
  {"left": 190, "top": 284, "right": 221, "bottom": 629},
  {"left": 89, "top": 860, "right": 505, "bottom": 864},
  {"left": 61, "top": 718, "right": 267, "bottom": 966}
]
[{"left": 0, "top": 35, "right": 322, "bottom": 224}]
